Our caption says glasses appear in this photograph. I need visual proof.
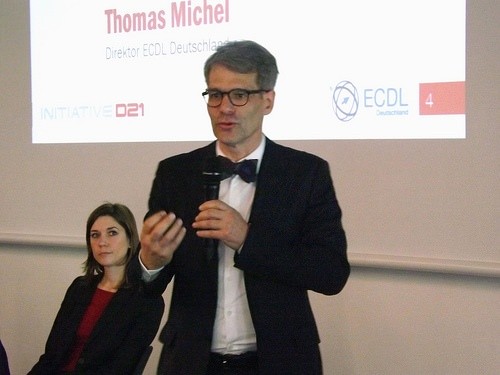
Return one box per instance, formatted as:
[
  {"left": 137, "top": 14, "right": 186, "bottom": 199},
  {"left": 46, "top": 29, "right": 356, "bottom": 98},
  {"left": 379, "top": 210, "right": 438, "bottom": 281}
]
[{"left": 201, "top": 87, "right": 264, "bottom": 107}]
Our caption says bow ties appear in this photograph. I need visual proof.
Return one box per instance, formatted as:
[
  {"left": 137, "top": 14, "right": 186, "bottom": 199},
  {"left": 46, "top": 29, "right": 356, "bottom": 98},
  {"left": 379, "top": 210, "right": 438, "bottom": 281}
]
[{"left": 214, "top": 155, "right": 258, "bottom": 184}]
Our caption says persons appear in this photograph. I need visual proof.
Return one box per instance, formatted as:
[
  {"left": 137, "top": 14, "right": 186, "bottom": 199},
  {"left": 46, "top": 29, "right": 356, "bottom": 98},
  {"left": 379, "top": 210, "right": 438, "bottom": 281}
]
[
  {"left": 127, "top": 40, "right": 350, "bottom": 375},
  {"left": 27, "top": 203, "right": 164, "bottom": 375}
]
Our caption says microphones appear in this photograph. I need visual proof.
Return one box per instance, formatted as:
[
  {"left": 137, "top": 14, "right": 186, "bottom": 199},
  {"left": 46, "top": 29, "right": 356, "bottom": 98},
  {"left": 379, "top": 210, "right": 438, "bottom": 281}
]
[{"left": 201, "top": 156, "right": 223, "bottom": 263}]
